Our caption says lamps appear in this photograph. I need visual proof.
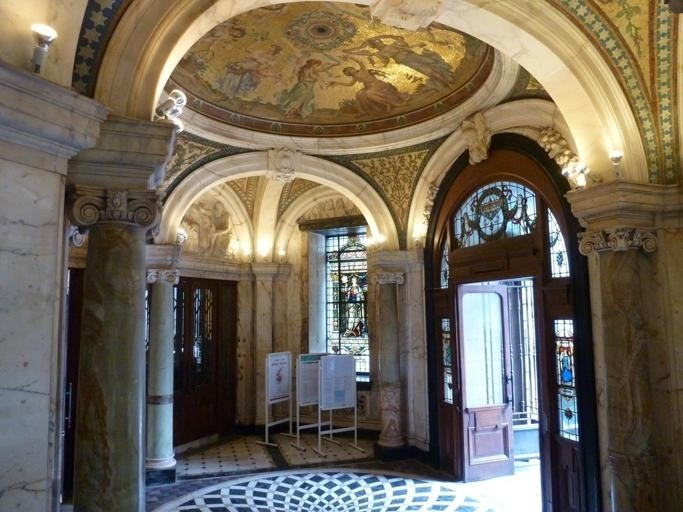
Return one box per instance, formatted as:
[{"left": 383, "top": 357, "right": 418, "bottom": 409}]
[
  {"left": 359, "top": 229, "right": 421, "bottom": 253},
  {"left": 156, "top": 93, "right": 184, "bottom": 135},
  {"left": 561, "top": 162, "right": 591, "bottom": 188},
  {"left": 29, "top": 23, "right": 56, "bottom": 74},
  {"left": 175, "top": 228, "right": 188, "bottom": 246},
  {"left": 610, "top": 151, "right": 623, "bottom": 177}
]
